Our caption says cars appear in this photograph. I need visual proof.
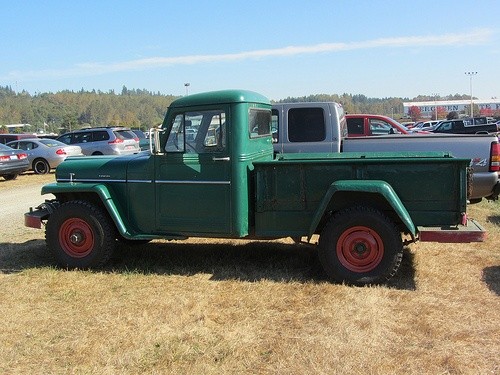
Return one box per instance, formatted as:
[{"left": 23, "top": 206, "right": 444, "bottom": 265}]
[
  {"left": 343, "top": 113, "right": 500, "bottom": 138},
  {"left": 5, "top": 137, "right": 86, "bottom": 175},
  {"left": 0, "top": 142, "right": 30, "bottom": 181},
  {"left": 0, "top": 125, "right": 199, "bottom": 181}
]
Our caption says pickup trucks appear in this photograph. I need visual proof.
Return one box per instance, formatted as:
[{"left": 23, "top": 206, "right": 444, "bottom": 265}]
[
  {"left": 215, "top": 100, "right": 500, "bottom": 205},
  {"left": 23, "top": 88, "right": 487, "bottom": 289}
]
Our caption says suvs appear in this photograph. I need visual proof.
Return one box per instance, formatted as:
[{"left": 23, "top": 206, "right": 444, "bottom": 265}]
[{"left": 55, "top": 125, "right": 142, "bottom": 156}]
